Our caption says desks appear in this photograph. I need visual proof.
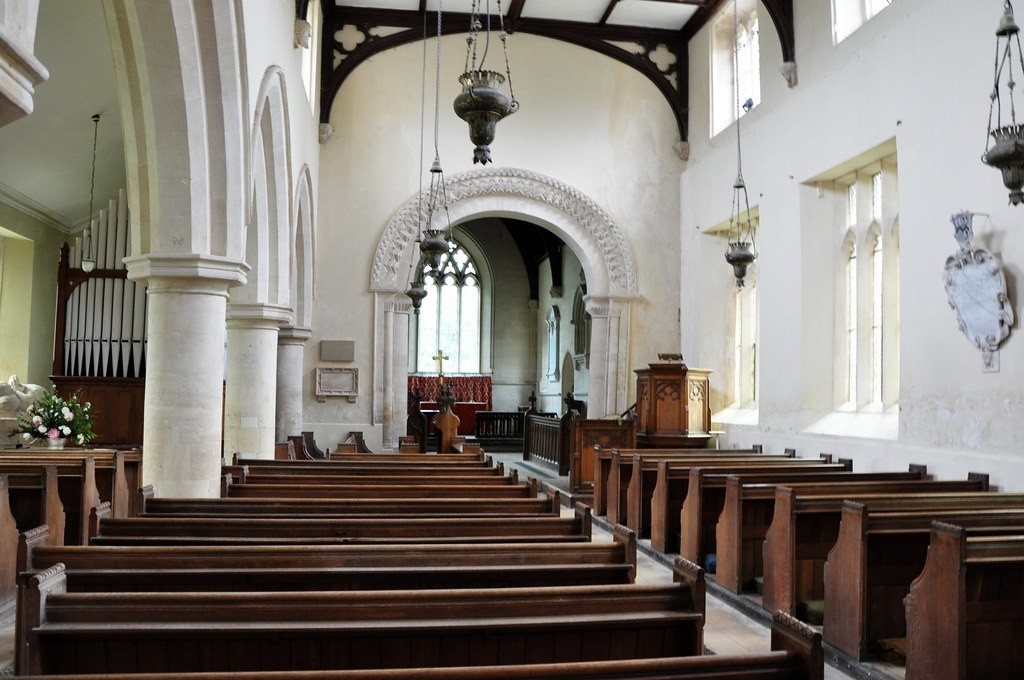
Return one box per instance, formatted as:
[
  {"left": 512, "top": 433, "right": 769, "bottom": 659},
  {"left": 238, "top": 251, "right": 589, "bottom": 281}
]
[{"left": 420, "top": 401, "right": 487, "bottom": 435}]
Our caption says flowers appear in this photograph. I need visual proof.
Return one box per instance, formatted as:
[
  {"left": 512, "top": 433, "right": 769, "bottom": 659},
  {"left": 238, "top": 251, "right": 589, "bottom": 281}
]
[{"left": 7, "top": 383, "right": 98, "bottom": 447}]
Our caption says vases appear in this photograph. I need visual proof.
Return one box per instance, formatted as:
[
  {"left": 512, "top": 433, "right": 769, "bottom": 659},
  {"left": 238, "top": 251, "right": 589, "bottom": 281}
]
[{"left": 46, "top": 438, "right": 68, "bottom": 450}]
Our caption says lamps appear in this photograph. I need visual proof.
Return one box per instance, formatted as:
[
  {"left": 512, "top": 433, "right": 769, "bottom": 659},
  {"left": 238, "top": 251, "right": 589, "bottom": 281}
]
[
  {"left": 81, "top": 112, "right": 101, "bottom": 274},
  {"left": 421, "top": 0, "right": 453, "bottom": 268},
  {"left": 453, "top": 0, "right": 522, "bottom": 167},
  {"left": 403, "top": 233, "right": 427, "bottom": 315},
  {"left": 979, "top": 0, "right": 1024, "bottom": 207},
  {"left": 724, "top": 0, "right": 759, "bottom": 288}
]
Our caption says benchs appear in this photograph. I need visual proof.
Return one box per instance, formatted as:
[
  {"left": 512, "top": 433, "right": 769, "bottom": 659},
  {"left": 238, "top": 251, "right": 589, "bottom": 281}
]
[{"left": 0, "top": 443, "right": 1024, "bottom": 680}]
